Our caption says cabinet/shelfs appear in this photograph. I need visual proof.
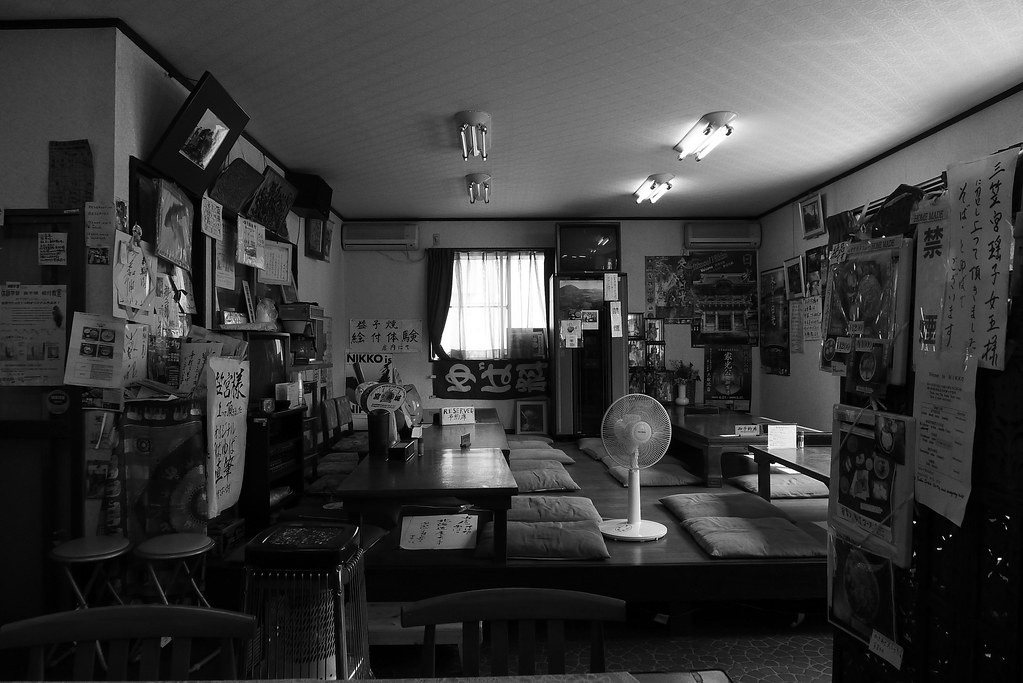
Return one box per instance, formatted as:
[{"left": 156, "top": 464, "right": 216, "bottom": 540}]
[{"left": 243, "top": 403, "right": 308, "bottom": 511}]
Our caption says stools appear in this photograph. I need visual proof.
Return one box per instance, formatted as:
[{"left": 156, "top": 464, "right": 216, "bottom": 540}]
[
  {"left": 42, "top": 536, "right": 144, "bottom": 673},
  {"left": 135, "top": 532, "right": 223, "bottom": 674}
]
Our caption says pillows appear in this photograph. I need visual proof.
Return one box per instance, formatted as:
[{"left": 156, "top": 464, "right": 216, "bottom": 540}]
[
  {"left": 473, "top": 430, "right": 830, "bottom": 561},
  {"left": 301, "top": 451, "right": 359, "bottom": 496}
]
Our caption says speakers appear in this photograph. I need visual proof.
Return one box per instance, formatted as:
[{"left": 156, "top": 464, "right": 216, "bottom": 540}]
[{"left": 285, "top": 172, "right": 333, "bottom": 221}]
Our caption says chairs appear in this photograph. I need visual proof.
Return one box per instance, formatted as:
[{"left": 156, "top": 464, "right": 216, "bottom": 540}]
[
  {"left": 0, "top": 604, "right": 258, "bottom": 682},
  {"left": 399, "top": 588, "right": 626, "bottom": 678},
  {"left": 321, "top": 394, "right": 369, "bottom": 455}
]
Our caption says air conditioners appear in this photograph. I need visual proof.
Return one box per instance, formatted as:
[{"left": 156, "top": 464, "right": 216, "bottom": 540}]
[
  {"left": 684, "top": 221, "right": 762, "bottom": 250},
  {"left": 341, "top": 222, "right": 418, "bottom": 251}
]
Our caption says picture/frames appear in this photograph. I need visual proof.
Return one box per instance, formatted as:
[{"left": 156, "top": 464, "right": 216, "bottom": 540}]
[
  {"left": 513, "top": 399, "right": 549, "bottom": 435},
  {"left": 782, "top": 255, "right": 806, "bottom": 300},
  {"left": 799, "top": 191, "right": 825, "bottom": 239},
  {"left": 245, "top": 165, "right": 298, "bottom": 233},
  {"left": 144, "top": 70, "right": 252, "bottom": 197},
  {"left": 305, "top": 217, "right": 327, "bottom": 260}
]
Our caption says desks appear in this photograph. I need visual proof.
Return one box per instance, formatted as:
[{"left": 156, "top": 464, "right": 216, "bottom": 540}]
[
  {"left": 412, "top": 403, "right": 510, "bottom": 461},
  {"left": 332, "top": 449, "right": 516, "bottom": 565},
  {"left": 745, "top": 445, "right": 831, "bottom": 505},
  {"left": 664, "top": 408, "right": 832, "bottom": 488}
]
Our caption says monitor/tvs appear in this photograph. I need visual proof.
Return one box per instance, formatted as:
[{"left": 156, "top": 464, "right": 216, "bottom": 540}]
[
  {"left": 556, "top": 222, "right": 622, "bottom": 272},
  {"left": 211, "top": 330, "right": 291, "bottom": 410}
]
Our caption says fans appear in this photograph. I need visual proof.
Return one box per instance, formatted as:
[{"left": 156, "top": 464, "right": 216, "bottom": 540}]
[{"left": 594, "top": 393, "right": 671, "bottom": 544}]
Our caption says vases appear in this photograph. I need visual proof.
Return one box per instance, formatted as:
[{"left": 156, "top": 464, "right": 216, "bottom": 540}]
[{"left": 675, "top": 382, "right": 689, "bottom": 406}]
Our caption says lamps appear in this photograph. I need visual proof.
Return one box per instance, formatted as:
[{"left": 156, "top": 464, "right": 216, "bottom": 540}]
[
  {"left": 465, "top": 173, "right": 492, "bottom": 204},
  {"left": 454, "top": 110, "right": 492, "bottom": 163}
]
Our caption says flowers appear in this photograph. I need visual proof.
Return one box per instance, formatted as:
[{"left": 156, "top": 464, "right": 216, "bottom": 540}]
[{"left": 667, "top": 358, "right": 702, "bottom": 384}]
